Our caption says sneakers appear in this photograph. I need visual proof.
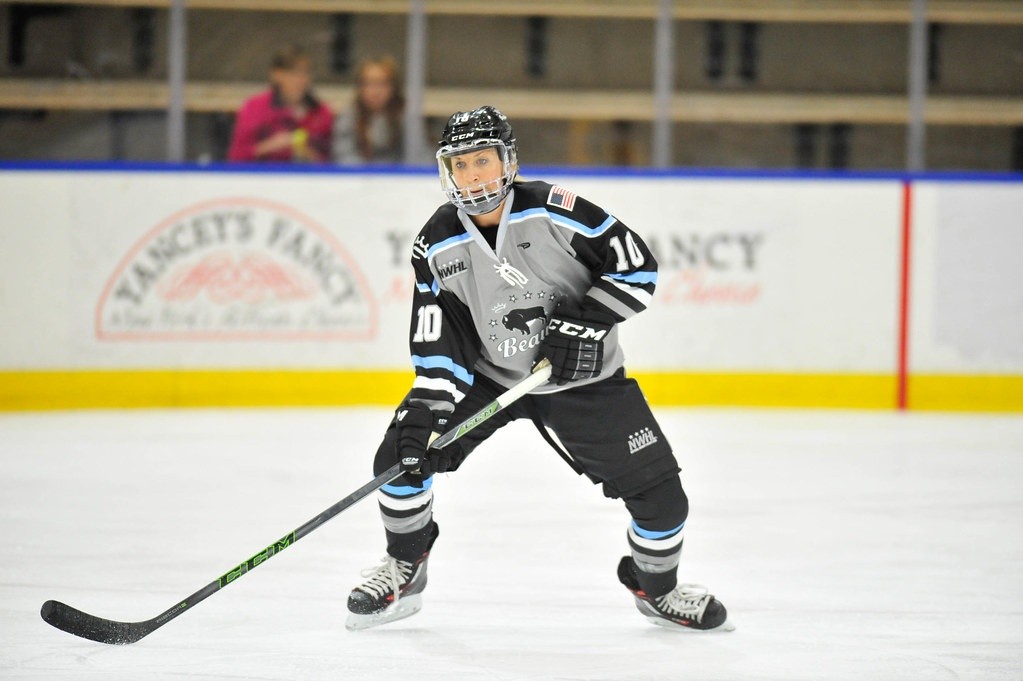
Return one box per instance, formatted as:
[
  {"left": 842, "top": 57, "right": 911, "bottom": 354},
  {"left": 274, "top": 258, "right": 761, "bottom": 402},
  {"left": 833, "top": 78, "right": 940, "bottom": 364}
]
[
  {"left": 617, "top": 557, "right": 734, "bottom": 634},
  {"left": 346, "top": 522, "right": 439, "bottom": 629}
]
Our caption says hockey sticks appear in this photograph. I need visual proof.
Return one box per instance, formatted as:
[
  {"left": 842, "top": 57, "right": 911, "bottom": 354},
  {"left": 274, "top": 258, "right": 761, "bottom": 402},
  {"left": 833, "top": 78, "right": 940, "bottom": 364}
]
[{"left": 37, "top": 360, "right": 555, "bottom": 649}]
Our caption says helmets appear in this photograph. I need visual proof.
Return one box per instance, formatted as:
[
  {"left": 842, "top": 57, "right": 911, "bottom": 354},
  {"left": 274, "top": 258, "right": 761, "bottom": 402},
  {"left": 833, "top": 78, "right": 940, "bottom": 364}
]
[{"left": 436, "top": 106, "right": 517, "bottom": 217}]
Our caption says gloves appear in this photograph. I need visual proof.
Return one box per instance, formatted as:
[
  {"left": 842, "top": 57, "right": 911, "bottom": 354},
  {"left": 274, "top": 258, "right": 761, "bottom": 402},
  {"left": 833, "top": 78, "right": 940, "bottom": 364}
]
[
  {"left": 530, "top": 312, "right": 611, "bottom": 386},
  {"left": 397, "top": 405, "right": 451, "bottom": 478}
]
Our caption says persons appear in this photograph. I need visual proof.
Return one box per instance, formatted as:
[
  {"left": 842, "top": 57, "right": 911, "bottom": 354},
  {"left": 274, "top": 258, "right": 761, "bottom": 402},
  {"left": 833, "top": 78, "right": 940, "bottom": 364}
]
[
  {"left": 230, "top": 44, "right": 334, "bottom": 163},
  {"left": 333, "top": 54, "right": 423, "bottom": 165},
  {"left": 334, "top": 104, "right": 733, "bottom": 632}
]
